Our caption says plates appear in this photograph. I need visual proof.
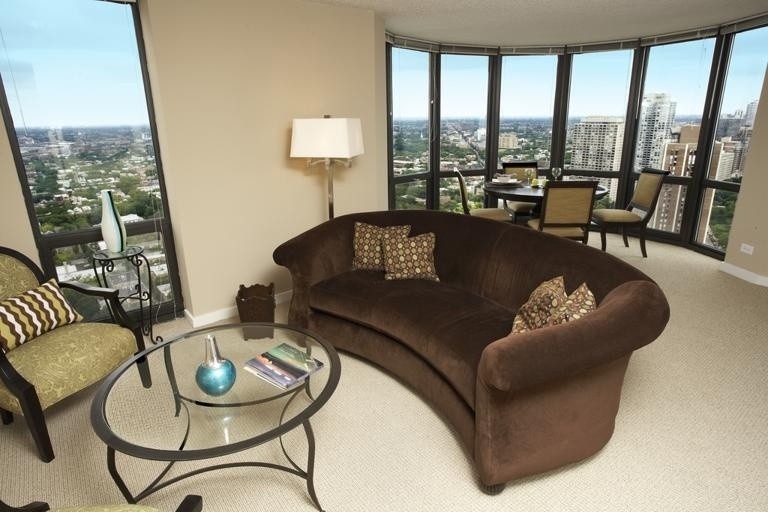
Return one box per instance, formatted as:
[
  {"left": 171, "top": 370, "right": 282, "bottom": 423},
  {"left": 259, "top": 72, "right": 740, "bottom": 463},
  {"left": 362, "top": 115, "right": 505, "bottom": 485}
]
[{"left": 493, "top": 178, "right": 521, "bottom": 185}]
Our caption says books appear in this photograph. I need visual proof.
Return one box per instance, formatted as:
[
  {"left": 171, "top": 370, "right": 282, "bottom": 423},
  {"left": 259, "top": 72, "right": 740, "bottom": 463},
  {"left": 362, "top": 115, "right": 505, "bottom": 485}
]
[
  {"left": 484, "top": 179, "right": 524, "bottom": 189},
  {"left": 242, "top": 343, "right": 324, "bottom": 390}
]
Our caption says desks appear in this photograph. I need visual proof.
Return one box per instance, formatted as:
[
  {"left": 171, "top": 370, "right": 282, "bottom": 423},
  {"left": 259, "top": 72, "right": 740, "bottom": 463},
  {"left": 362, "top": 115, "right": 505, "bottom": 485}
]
[{"left": 482, "top": 183, "right": 610, "bottom": 219}]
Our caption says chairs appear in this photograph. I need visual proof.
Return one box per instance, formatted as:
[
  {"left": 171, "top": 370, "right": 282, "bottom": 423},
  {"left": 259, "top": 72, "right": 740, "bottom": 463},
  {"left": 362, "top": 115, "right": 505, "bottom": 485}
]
[
  {"left": 502, "top": 161, "right": 538, "bottom": 224},
  {"left": 455, "top": 170, "right": 512, "bottom": 222},
  {"left": 0, "top": 246, "right": 152, "bottom": 463},
  {"left": 591, "top": 167, "right": 671, "bottom": 257},
  {"left": 0, "top": 495, "right": 203, "bottom": 512},
  {"left": 527, "top": 181, "right": 599, "bottom": 244}
]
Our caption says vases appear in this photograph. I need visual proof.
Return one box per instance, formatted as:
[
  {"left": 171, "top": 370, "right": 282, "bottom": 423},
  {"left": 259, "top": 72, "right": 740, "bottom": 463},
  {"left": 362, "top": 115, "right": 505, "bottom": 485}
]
[{"left": 101, "top": 190, "right": 126, "bottom": 252}]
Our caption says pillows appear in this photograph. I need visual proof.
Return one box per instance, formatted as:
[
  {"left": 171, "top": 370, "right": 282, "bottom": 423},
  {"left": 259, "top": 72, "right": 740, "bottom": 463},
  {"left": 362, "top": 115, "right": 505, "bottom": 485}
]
[
  {"left": 549, "top": 281, "right": 596, "bottom": 327},
  {"left": 353, "top": 222, "right": 411, "bottom": 272},
  {"left": 382, "top": 232, "right": 440, "bottom": 282},
  {"left": 512, "top": 276, "right": 567, "bottom": 332},
  {"left": 0, "top": 277, "right": 84, "bottom": 354}
]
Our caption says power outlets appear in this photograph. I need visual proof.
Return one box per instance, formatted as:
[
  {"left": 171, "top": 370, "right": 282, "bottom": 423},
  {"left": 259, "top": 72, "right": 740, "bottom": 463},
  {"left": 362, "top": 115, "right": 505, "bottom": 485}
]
[{"left": 740, "top": 243, "right": 754, "bottom": 255}]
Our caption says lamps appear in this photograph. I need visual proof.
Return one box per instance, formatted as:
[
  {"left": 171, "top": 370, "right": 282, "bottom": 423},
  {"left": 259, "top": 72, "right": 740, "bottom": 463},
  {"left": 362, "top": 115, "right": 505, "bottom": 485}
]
[{"left": 290, "top": 115, "right": 365, "bottom": 219}]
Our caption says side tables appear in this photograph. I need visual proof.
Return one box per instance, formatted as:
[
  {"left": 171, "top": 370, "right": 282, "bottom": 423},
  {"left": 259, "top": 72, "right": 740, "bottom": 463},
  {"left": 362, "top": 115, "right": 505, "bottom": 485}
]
[{"left": 93, "top": 246, "right": 163, "bottom": 344}]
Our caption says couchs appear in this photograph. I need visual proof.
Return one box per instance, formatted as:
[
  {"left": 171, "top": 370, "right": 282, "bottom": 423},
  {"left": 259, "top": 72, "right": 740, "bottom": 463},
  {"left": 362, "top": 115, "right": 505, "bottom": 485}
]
[{"left": 273, "top": 210, "right": 670, "bottom": 495}]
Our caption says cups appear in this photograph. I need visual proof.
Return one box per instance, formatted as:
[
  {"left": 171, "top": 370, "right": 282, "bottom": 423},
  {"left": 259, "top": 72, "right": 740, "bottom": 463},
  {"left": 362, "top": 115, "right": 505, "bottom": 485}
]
[
  {"left": 525, "top": 168, "right": 534, "bottom": 187},
  {"left": 498, "top": 175, "right": 510, "bottom": 180},
  {"left": 552, "top": 167, "right": 561, "bottom": 181}
]
